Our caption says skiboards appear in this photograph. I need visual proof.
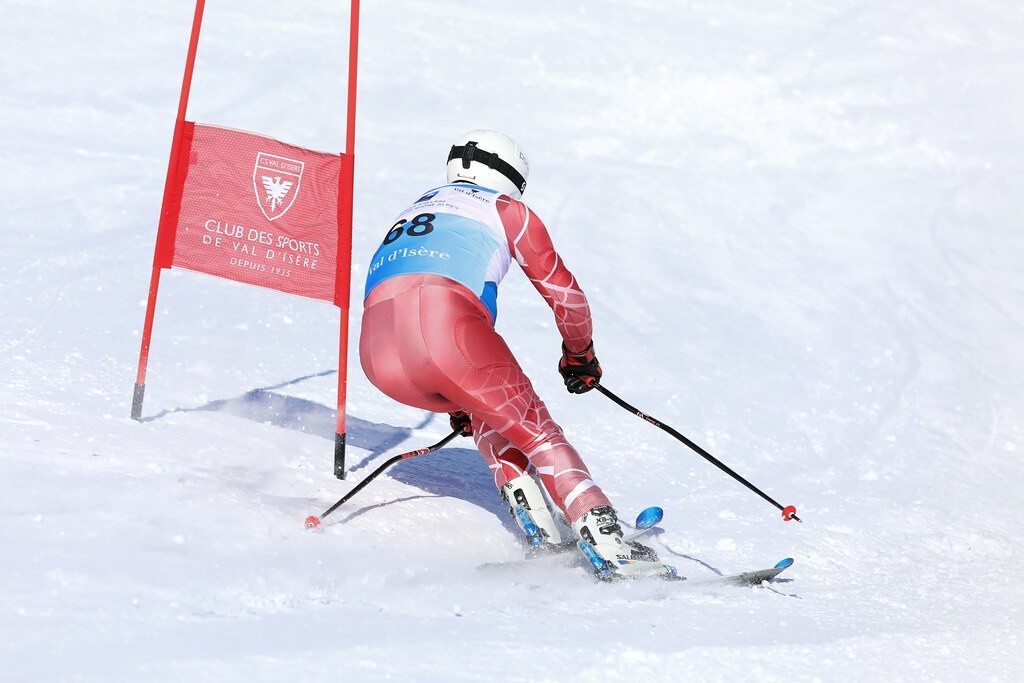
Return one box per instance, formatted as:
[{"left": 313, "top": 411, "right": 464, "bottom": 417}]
[{"left": 473, "top": 506, "right": 794, "bottom": 594}]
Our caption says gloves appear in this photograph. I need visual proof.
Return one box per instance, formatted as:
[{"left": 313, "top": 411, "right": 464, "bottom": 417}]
[
  {"left": 558, "top": 339, "right": 602, "bottom": 394},
  {"left": 448, "top": 410, "right": 473, "bottom": 437}
]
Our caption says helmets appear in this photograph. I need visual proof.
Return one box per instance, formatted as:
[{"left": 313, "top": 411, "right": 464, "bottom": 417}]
[{"left": 446, "top": 130, "right": 529, "bottom": 200}]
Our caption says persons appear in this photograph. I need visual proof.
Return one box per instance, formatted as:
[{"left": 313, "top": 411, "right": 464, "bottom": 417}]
[{"left": 360, "top": 131, "right": 680, "bottom": 580}]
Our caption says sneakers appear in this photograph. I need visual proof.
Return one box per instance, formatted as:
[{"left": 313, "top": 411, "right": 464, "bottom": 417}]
[
  {"left": 571, "top": 505, "right": 687, "bottom": 582},
  {"left": 499, "top": 474, "right": 580, "bottom": 558}
]
